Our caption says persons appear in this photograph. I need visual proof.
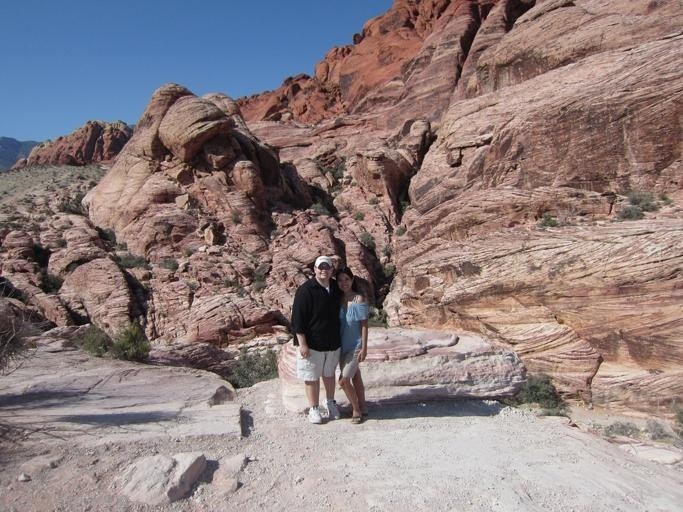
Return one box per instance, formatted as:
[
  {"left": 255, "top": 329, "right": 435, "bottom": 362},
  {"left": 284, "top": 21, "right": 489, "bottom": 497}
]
[
  {"left": 288, "top": 256, "right": 344, "bottom": 425},
  {"left": 336, "top": 268, "right": 369, "bottom": 423}
]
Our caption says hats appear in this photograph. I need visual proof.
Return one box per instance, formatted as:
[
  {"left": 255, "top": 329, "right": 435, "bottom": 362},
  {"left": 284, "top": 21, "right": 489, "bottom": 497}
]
[{"left": 315, "top": 255, "right": 332, "bottom": 268}]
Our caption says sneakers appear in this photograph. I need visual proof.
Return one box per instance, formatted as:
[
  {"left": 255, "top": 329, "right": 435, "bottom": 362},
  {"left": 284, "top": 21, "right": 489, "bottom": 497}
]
[
  {"left": 322, "top": 398, "right": 341, "bottom": 420},
  {"left": 309, "top": 406, "right": 322, "bottom": 423}
]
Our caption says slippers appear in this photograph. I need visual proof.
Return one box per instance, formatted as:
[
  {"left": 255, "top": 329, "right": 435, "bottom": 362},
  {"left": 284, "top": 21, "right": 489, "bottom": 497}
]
[{"left": 350, "top": 414, "right": 368, "bottom": 424}]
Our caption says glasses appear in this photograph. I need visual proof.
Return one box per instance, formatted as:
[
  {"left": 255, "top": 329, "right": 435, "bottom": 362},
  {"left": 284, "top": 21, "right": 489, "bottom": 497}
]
[{"left": 319, "top": 264, "right": 331, "bottom": 270}]
